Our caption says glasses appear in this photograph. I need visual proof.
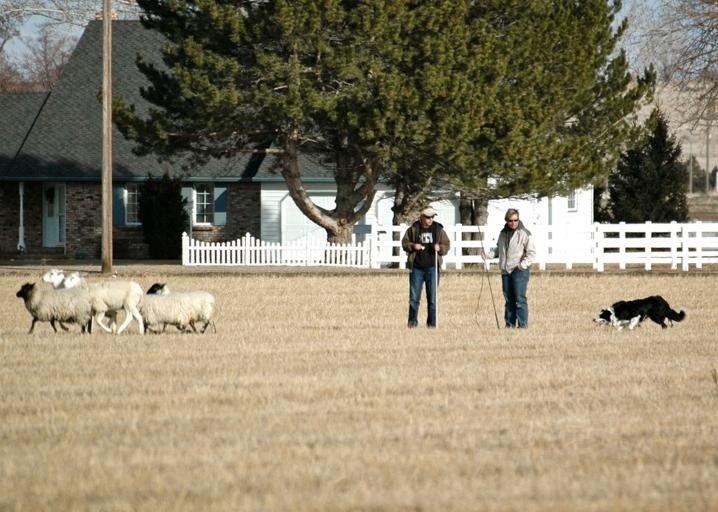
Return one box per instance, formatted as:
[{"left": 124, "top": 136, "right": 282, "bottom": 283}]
[{"left": 508, "top": 219, "right": 519, "bottom": 222}]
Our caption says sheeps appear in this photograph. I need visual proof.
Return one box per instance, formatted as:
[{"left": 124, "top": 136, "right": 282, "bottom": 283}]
[{"left": 16, "top": 269, "right": 217, "bottom": 336}]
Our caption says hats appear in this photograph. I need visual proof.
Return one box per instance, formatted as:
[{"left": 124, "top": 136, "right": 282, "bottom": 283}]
[{"left": 420, "top": 206, "right": 438, "bottom": 217}]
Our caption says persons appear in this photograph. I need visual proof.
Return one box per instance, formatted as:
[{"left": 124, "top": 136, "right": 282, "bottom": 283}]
[
  {"left": 480, "top": 208, "right": 539, "bottom": 329},
  {"left": 401, "top": 205, "right": 451, "bottom": 327}
]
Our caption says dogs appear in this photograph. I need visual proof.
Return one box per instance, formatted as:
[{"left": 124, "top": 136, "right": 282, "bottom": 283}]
[{"left": 593, "top": 295, "right": 686, "bottom": 332}]
